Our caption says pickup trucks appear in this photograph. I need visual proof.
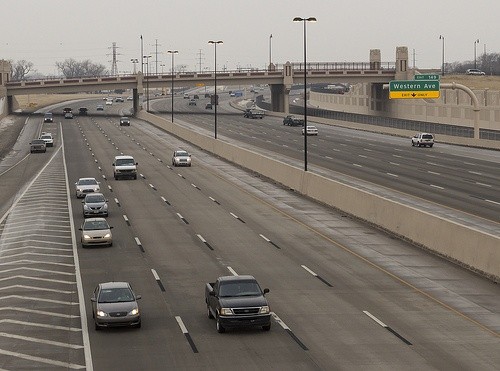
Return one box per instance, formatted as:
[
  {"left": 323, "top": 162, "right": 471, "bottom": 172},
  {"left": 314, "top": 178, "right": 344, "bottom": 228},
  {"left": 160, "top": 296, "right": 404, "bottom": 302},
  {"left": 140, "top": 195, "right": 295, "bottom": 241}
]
[{"left": 204, "top": 274, "right": 272, "bottom": 333}]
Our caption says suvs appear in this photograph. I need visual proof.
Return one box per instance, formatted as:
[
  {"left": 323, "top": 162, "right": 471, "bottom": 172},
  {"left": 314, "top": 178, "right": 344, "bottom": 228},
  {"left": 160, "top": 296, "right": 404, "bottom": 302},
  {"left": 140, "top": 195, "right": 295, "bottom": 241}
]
[
  {"left": 29, "top": 139, "right": 47, "bottom": 153},
  {"left": 411, "top": 133, "right": 434, "bottom": 148},
  {"left": 39, "top": 135, "right": 54, "bottom": 147}
]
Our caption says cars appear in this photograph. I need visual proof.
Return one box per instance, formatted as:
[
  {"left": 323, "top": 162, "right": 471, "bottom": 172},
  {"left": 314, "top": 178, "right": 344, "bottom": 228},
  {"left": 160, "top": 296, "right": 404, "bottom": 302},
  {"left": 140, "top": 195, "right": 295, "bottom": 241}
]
[
  {"left": 97, "top": 96, "right": 133, "bottom": 111},
  {"left": 78, "top": 217, "right": 114, "bottom": 248},
  {"left": 81, "top": 193, "right": 109, "bottom": 217},
  {"left": 75, "top": 177, "right": 101, "bottom": 198},
  {"left": 79, "top": 107, "right": 88, "bottom": 115},
  {"left": 302, "top": 125, "right": 318, "bottom": 136},
  {"left": 183, "top": 84, "right": 268, "bottom": 119},
  {"left": 172, "top": 150, "right": 192, "bottom": 167},
  {"left": 120, "top": 116, "right": 130, "bottom": 126},
  {"left": 90, "top": 279, "right": 142, "bottom": 331},
  {"left": 63, "top": 107, "right": 73, "bottom": 119}
]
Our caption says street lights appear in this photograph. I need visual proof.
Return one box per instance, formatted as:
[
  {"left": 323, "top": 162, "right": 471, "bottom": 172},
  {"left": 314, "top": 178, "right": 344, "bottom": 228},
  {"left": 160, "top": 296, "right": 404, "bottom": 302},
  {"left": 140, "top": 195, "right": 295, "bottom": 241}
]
[
  {"left": 208, "top": 40, "right": 224, "bottom": 139},
  {"left": 168, "top": 50, "right": 179, "bottom": 123},
  {"left": 144, "top": 55, "right": 152, "bottom": 112},
  {"left": 291, "top": 17, "right": 318, "bottom": 172}
]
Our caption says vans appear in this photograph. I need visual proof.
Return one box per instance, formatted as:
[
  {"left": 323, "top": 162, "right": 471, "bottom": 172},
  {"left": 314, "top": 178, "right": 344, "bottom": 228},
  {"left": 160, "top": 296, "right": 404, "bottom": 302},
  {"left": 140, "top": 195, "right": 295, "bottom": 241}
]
[
  {"left": 44, "top": 113, "right": 53, "bottom": 123},
  {"left": 112, "top": 155, "right": 139, "bottom": 180}
]
